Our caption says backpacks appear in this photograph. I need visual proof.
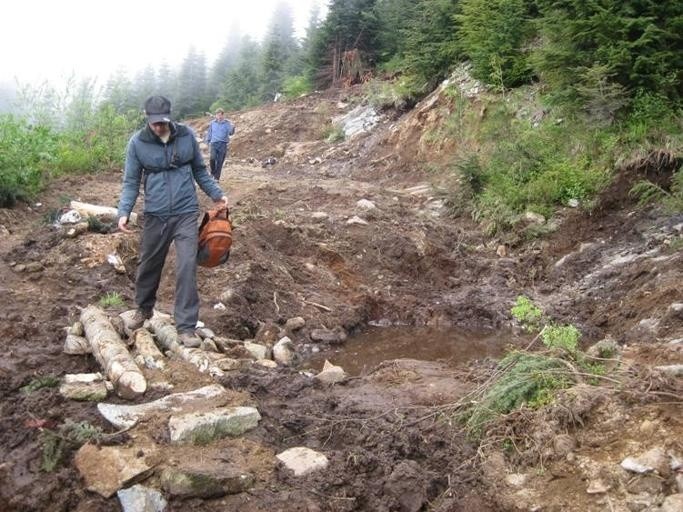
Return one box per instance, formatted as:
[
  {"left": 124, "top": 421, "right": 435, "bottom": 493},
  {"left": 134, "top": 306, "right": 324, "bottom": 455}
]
[{"left": 196, "top": 203, "right": 233, "bottom": 267}]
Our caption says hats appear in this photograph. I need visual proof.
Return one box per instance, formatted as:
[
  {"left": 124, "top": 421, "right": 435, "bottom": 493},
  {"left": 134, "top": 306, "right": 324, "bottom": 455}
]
[{"left": 144, "top": 95, "right": 171, "bottom": 124}]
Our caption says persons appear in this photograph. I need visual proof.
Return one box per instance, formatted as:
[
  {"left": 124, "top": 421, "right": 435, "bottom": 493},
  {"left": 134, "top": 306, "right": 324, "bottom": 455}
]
[
  {"left": 117, "top": 96, "right": 229, "bottom": 348},
  {"left": 205, "top": 107, "right": 236, "bottom": 183}
]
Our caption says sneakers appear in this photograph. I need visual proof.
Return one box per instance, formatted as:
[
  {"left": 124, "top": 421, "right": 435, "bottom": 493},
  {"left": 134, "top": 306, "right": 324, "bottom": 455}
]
[
  {"left": 176, "top": 332, "right": 201, "bottom": 348},
  {"left": 128, "top": 306, "right": 154, "bottom": 329},
  {"left": 215, "top": 179, "right": 219, "bottom": 182}
]
